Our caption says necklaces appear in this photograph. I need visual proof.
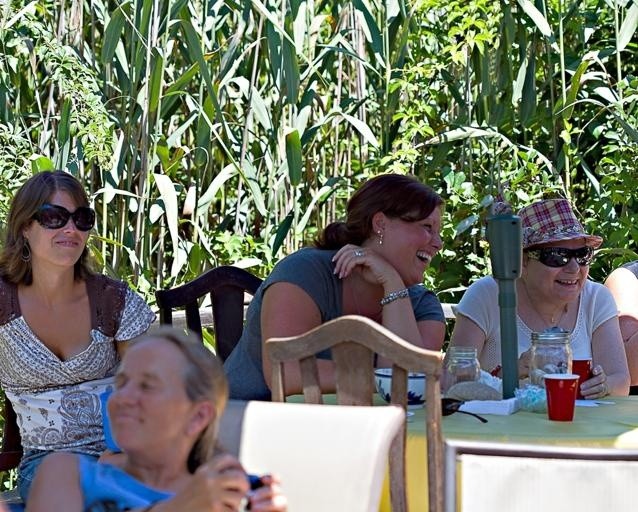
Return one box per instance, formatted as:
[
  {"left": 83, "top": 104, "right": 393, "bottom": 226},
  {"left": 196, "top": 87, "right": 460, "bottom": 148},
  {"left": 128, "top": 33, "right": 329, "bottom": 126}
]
[
  {"left": 524, "top": 306, "right": 564, "bottom": 324},
  {"left": 521, "top": 277, "right": 564, "bottom": 329},
  {"left": 351, "top": 274, "right": 383, "bottom": 321}
]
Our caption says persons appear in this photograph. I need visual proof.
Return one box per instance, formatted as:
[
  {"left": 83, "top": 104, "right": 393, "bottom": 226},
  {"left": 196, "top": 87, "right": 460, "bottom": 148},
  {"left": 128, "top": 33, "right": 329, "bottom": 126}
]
[
  {"left": 27, "top": 326, "right": 289, "bottom": 512},
  {"left": 442, "top": 198, "right": 631, "bottom": 400},
  {"left": 603, "top": 259, "right": 637, "bottom": 386},
  {"left": 0, "top": 170, "right": 158, "bottom": 512},
  {"left": 222, "top": 175, "right": 446, "bottom": 400}
]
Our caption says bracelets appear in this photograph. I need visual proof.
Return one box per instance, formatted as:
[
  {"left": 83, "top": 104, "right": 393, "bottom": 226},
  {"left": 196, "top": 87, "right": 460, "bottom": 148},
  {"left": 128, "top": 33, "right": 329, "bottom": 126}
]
[{"left": 380, "top": 289, "right": 410, "bottom": 306}]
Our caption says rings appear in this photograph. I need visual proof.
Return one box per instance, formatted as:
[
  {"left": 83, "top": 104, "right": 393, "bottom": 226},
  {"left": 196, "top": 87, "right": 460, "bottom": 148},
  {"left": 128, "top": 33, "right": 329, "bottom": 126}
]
[{"left": 354, "top": 249, "right": 364, "bottom": 256}]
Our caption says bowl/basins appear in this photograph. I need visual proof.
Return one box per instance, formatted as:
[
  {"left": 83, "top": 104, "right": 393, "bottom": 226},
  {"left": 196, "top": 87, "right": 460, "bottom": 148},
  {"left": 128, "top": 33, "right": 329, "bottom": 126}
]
[{"left": 373, "top": 366, "right": 428, "bottom": 408}]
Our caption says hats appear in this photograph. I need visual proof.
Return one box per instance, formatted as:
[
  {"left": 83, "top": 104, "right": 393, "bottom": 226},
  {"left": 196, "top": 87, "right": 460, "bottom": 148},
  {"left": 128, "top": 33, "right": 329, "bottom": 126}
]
[{"left": 515, "top": 198, "right": 602, "bottom": 247}]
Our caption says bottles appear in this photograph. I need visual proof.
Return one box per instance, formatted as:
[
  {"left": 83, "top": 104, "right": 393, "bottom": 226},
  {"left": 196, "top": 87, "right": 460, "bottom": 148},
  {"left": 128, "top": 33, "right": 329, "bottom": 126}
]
[
  {"left": 444, "top": 346, "right": 482, "bottom": 397},
  {"left": 527, "top": 330, "right": 573, "bottom": 388}
]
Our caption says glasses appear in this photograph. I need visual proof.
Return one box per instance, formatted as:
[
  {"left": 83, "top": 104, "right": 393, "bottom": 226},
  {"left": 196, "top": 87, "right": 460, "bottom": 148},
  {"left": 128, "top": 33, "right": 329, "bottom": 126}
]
[
  {"left": 528, "top": 245, "right": 593, "bottom": 268},
  {"left": 31, "top": 204, "right": 96, "bottom": 234}
]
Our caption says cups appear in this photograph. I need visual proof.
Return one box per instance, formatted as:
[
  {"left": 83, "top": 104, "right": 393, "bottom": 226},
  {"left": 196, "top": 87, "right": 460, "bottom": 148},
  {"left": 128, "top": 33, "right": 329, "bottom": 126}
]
[
  {"left": 571, "top": 358, "right": 592, "bottom": 400},
  {"left": 543, "top": 372, "right": 581, "bottom": 422}
]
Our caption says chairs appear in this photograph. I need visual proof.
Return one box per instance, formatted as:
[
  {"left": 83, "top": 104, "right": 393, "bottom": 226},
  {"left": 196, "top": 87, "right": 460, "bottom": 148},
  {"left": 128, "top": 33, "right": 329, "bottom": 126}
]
[
  {"left": 0, "top": 392, "right": 26, "bottom": 512},
  {"left": 444, "top": 437, "right": 638, "bottom": 512},
  {"left": 155, "top": 265, "right": 265, "bottom": 366},
  {"left": 216, "top": 400, "right": 407, "bottom": 512},
  {"left": 265, "top": 315, "right": 445, "bottom": 512}
]
